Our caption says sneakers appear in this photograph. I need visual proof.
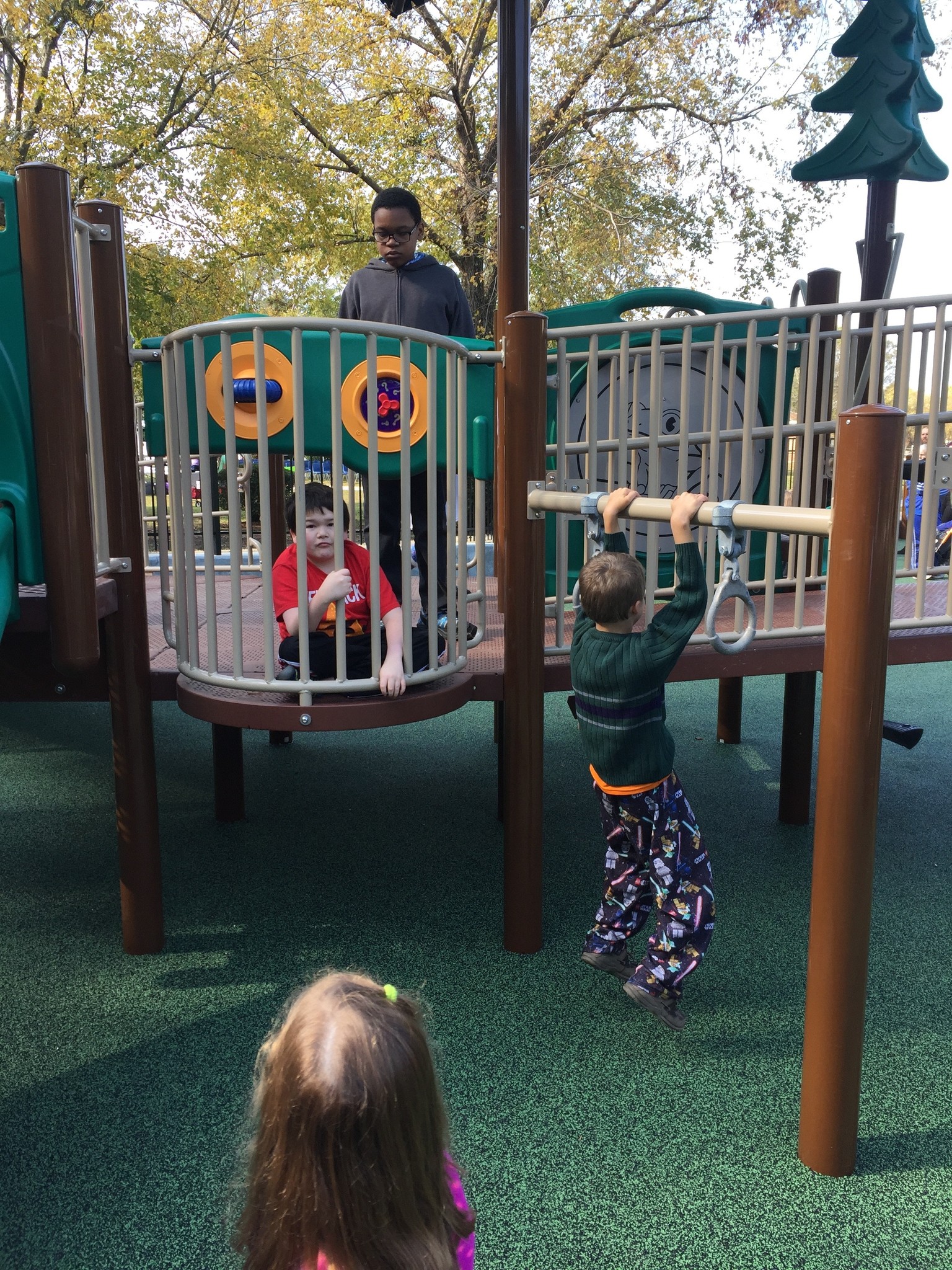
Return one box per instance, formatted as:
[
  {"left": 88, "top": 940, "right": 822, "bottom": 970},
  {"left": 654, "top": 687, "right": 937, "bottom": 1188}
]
[
  {"left": 581, "top": 948, "right": 638, "bottom": 980},
  {"left": 416, "top": 608, "right": 477, "bottom": 640},
  {"left": 623, "top": 979, "right": 685, "bottom": 1031}
]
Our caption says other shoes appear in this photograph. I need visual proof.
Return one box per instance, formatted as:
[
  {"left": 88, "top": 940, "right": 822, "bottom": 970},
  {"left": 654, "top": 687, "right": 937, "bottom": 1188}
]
[
  {"left": 930, "top": 572, "right": 945, "bottom": 578},
  {"left": 897, "top": 547, "right": 906, "bottom": 554},
  {"left": 276, "top": 666, "right": 300, "bottom": 682},
  {"left": 913, "top": 572, "right": 932, "bottom": 580}
]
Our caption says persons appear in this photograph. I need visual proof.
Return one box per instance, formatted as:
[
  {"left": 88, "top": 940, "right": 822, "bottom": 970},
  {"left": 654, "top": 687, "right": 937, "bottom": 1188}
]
[
  {"left": 897, "top": 425, "right": 930, "bottom": 555},
  {"left": 231, "top": 967, "right": 475, "bottom": 1270},
  {"left": 569, "top": 489, "right": 715, "bottom": 1032},
  {"left": 339, "top": 187, "right": 486, "bottom": 651},
  {"left": 904, "top": 444, "right": 946, "bottom": 579},
  {"left": 272, "top": 481, "right": 446, "bottom": 705},
  {"left": 934, "top": 491, "right": 952, "bottom": 568}
]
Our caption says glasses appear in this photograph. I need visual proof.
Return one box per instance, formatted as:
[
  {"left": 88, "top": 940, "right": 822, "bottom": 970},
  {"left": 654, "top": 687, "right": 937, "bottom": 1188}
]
[{"left": 372, "top": 221, "right": 420, "bottom": 244}]
